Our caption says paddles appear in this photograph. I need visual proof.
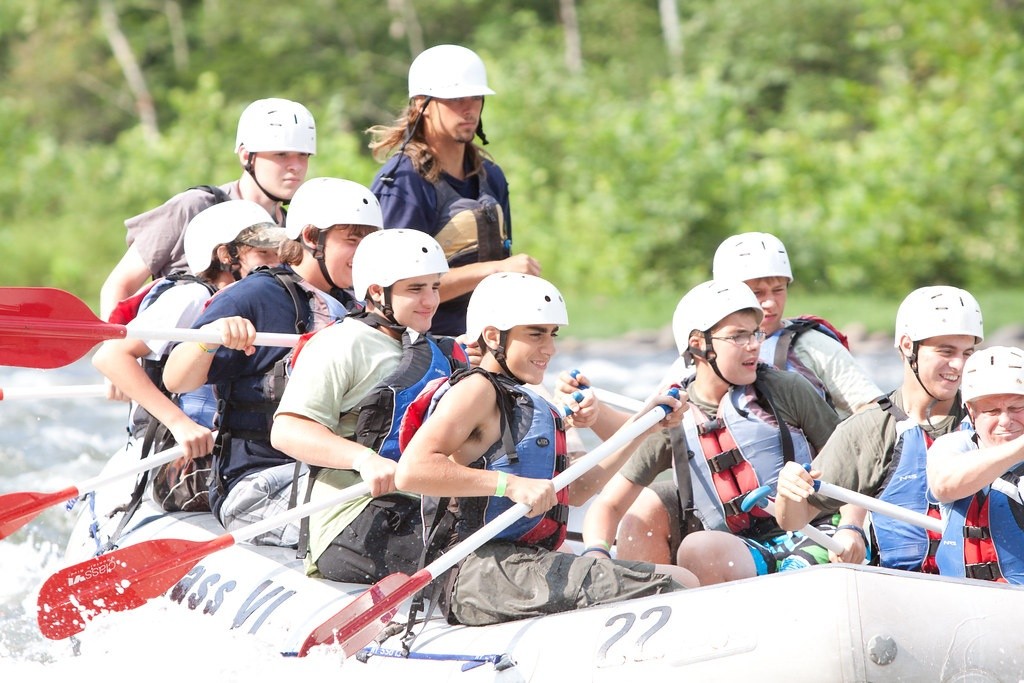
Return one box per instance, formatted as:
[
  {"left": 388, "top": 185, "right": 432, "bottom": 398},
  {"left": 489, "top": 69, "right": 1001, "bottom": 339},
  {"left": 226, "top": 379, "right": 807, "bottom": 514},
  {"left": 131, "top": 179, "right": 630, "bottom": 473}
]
[
  {"left": 569, "top": 369, "right": 644, "bottom": 411},
  {"left": 741, "top": 485, "right": 870, "bottom": 565},
  {"left": 37, "top": 391, "right": 584, "bottom": 640},
  {"left": 803, "top": 462, "right": 941, "bottom": 532},
  {"left": 0, "top": 384, "right": 107, "bottom": 400},
  {"left": 0, "top": 287, "right": 467, "bottom": 369},
  {"left": 990, "top": 476, "right": 1024, "bottom": 506},
  {"left": 298, "top": 386, "right": 680, "bottom": 659},
  {"left": 0, "top": 429, "right": 220, "bottom": 541}
]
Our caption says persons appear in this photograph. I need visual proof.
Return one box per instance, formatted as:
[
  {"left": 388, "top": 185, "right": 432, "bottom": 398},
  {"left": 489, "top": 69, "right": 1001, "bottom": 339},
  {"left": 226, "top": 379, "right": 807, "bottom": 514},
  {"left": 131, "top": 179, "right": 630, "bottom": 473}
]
[
  {"left": 91, "top": 44, "right": 542, "bottom": 585},
  {"left": 776, "top": 285, "right": 984, "bottom": 574},
  {"left": 395, "top": 271, "right": 702, "bottom": 625},
  {"left": 581, "top": 279, "right": 878, "bottom": 587},
  {"left": 926, "top": 346, "right": 1024, "bottom": 585},
  {"left": 553, "top": 232, "right": 893, "bottom": 579}
]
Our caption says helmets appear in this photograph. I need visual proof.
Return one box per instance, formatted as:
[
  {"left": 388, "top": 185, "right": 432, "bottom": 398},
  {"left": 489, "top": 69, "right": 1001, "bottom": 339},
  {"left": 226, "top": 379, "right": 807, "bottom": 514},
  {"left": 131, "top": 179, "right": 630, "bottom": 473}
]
[
  {"left": 286, "top": 177, "right": 384, "bottom": 240},
  {"left": 184, "top": 200, "right": 286, "bottom": 277},
  {"left": 961, "top": 346, "right": 1024, "bottom": 409},
  {"left": 234, "top": 98, "right": 317, "bottom": 156},
  {"left": 351, "top": 229, "right": 449, "bottom": 302},
  {"left": 463, "top": 272, "right": 569, "bottom": 346},
  {"left": 672, "top": 280, "right": 765, "bottom": 356},
  {"left": 713, "top": 232, "right": 794, "bottom": 284},
  {"left": 894, "top": 286, "right": 985, "bottom": 349},
  {"left": 408, "top": 45, "right": 496, "bottom": 100}
]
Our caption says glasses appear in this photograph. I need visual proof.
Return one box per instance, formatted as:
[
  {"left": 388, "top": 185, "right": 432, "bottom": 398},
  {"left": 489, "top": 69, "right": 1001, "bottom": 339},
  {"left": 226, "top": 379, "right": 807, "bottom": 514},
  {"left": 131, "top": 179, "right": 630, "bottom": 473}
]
[{"left": 701, "top": 331, "right": 767, "bottom": 345}]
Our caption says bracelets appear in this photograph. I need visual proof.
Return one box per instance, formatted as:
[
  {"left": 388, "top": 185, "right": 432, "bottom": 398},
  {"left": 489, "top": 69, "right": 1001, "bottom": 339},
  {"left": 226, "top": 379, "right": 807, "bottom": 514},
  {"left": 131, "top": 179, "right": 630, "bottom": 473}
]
[
  {"left": 835, "top": 525, "right": 868, "bottom": 548},
  {"left": 351, "top": 448, "right": 375, "bottom": 471},
  {"left": 581, "top": 547, "right": 611, "bottom": 559},
  {"left": 585, "top": 539, "right": 610, "bottom": 552},
  {"left": 492, "top": 469, "right": 507, "bottom": 497},
  {"left": 194, "top": 340, "right": 219, "bottom": 353}
]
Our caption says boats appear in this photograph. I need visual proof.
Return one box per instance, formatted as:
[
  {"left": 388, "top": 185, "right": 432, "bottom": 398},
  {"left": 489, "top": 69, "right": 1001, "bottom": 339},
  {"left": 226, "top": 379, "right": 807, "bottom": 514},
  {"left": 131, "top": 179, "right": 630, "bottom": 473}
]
[{"left": 60, "top": 370, "right": 1023, "bottom": 681}]
